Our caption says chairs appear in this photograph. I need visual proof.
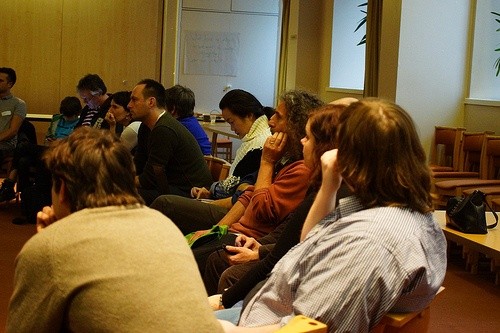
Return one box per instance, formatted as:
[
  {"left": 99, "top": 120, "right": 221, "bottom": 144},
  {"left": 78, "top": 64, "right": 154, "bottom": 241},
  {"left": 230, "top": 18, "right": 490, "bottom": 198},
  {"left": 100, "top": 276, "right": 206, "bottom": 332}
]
[
  {"left": 426, "top": 125, "right": 500, "bottom": 215},
  {"left": 203, "top": 156, "right": 231, "bottom": 184}
]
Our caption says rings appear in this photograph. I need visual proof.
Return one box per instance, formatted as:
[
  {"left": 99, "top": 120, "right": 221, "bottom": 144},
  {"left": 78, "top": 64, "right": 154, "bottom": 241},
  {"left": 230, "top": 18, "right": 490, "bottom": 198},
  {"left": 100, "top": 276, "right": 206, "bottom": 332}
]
[{"left": 270, "top": 141, "right": 274, "bottom": 143}]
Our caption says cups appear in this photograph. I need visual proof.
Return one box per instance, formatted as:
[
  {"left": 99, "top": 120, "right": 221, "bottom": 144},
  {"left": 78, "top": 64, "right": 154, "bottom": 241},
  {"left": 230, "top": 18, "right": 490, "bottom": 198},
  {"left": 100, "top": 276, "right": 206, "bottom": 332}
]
[
  {"left": 210, "top": 115, "right": 216, "bottom": 124},
  {"left": 203, "top": 113, "right": 210, "bottom": 122}
]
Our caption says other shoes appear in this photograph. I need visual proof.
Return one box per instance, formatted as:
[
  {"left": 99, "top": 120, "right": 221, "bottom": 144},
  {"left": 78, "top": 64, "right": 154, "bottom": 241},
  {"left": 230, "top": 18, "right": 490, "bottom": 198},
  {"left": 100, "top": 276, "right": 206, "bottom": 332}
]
[
  {"left": 0, "top": 181, "right": 16, "bottom": 203},
  {"left": 13, "top": 217, "right": 37, "bottom": 225}
]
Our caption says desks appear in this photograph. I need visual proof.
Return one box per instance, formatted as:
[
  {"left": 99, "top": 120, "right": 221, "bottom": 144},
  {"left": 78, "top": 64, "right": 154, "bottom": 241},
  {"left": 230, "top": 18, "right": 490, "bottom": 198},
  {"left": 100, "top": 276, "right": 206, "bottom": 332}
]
[{"left": 427, "top": 211, "right": 500, "bottom": 285}]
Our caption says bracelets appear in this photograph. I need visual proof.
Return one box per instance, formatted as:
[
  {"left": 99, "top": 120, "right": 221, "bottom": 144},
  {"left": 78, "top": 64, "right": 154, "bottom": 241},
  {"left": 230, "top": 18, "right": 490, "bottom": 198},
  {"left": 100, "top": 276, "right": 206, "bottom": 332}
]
[{"left": 218, "top": 288, "right": 228, "bottom": 310}]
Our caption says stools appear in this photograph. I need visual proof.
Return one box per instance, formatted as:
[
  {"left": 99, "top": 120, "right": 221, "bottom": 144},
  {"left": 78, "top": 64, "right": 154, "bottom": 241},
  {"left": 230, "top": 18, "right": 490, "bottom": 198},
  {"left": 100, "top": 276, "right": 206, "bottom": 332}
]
[{"left": 207, "top": 139, "right": 232, "bottom": 163}]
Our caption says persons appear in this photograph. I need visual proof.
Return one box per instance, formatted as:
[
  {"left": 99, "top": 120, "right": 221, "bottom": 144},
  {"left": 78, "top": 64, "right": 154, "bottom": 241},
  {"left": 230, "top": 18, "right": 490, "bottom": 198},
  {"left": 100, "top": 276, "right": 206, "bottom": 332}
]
[{"left": 0, "top": 66, "right": 448, "bottom": 333}]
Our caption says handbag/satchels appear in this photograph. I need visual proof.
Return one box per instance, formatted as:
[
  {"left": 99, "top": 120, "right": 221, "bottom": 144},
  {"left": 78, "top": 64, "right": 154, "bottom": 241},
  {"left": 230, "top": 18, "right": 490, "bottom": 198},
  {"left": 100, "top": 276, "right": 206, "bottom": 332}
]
[{"left": 445, "top": 189, "right": 499, "bottom": 235}]
